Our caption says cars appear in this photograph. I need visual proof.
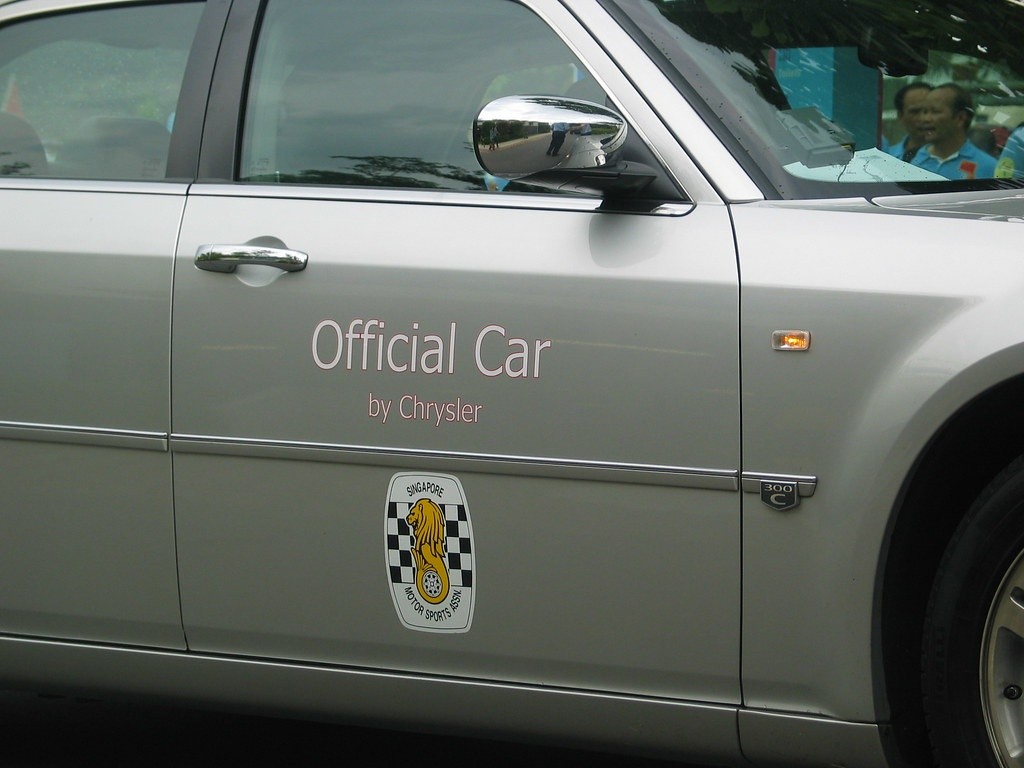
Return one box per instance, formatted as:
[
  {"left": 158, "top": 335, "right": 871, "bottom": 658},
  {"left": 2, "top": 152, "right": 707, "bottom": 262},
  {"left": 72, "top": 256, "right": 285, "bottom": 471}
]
[{"left": 2, "top": 2, "right": 1024, "bottom": 766}]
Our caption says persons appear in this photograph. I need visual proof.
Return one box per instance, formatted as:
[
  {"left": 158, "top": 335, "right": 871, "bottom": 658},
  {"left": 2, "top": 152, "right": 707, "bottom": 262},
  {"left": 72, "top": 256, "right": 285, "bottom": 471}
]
[{"left": 883, "top": 81, "right": 1024, "bottom": 178}]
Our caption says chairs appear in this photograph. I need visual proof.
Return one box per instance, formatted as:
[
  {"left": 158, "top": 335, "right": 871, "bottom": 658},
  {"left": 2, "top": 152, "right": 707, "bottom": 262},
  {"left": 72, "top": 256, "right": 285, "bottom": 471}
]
[
  {"left": 0, "top": 110, "right": 50, "bottom": 176},
  {"left": 54, "top": 116, "right": 172, "bottom": 183}
]
